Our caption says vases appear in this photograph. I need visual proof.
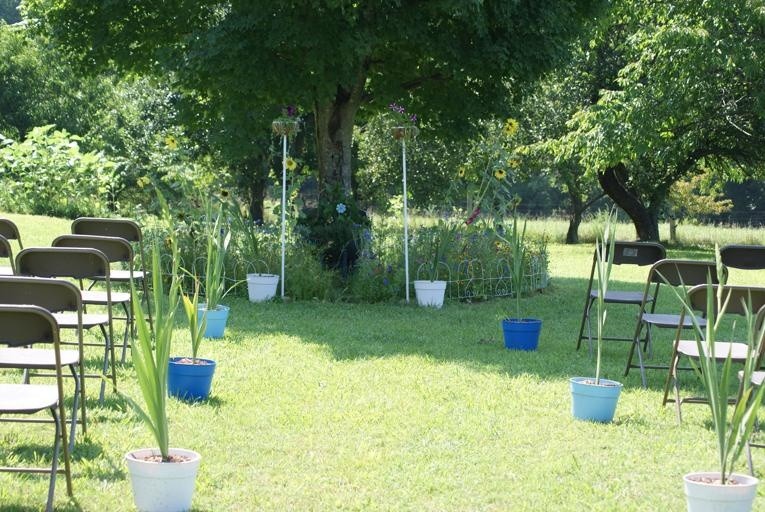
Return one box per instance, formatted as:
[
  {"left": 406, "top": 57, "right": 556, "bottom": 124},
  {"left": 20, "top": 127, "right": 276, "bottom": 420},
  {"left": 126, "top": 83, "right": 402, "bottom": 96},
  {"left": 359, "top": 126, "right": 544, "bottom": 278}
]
[
  {"left": 276, "top": 124, "right": 296, "bottom": 133},
  {"left": 392, "top": 129, "right": 413, "bottom": 138}
]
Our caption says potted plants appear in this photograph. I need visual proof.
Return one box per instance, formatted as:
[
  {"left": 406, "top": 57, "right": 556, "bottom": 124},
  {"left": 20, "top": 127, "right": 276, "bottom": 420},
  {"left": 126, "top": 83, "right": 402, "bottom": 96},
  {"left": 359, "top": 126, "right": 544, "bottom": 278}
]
[
  {"left": 570, "top": 203, "right": 622, "bottom": 422},
  {"left": 495, "top": 201, "right": 542, "bottom": 351},
  {"left": 413, "top": 241, "right": 447, "bottom": 309}
]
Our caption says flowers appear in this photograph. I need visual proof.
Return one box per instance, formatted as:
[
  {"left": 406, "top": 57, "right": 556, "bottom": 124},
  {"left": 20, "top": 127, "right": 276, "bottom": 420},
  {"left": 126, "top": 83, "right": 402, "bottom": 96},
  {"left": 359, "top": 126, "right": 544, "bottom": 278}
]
[
  {"left": 271, "top": 104, "right": 303, "bottom": 147},
  {"left": 388, "top": 103, "right": 417, "bottom": 144}
]
